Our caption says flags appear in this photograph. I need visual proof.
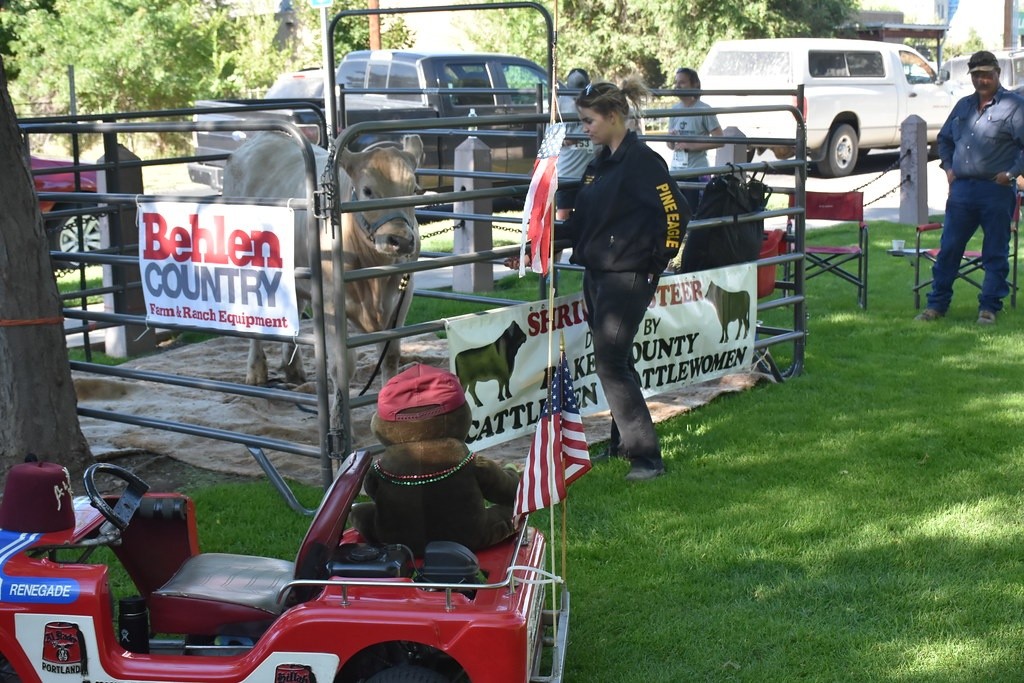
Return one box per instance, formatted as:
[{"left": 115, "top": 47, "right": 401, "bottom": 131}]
[{"left": 511, "top": 117, "right": 590, "bottom": 523}]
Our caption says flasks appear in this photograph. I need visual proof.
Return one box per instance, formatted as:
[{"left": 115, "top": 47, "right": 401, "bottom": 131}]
[{"left": 118, "top": 595, "right": 150, "bottom": 654}]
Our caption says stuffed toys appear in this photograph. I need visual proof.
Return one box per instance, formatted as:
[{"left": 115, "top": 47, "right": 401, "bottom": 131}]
[{"left": 359, "top": 363, "right": 526, "bottom": 556}]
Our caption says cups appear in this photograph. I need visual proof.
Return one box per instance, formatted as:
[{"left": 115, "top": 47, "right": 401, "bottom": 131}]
[{"left": 891, "top": 240, "right": 905, "bottom": 249}]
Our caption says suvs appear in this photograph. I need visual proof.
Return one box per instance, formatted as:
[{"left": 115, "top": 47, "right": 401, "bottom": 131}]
[{"left": 937, "top": 46, "right": 1024, "bottom": 103}]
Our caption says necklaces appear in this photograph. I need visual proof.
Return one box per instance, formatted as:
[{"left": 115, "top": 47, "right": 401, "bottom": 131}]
[{"left": 372, "top": 450, "right": 477, "bottom": 485}]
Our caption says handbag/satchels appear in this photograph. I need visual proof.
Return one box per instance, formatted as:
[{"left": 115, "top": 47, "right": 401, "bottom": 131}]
[{"left": 678, "top": 162, "right": 773, "bottom": 274}]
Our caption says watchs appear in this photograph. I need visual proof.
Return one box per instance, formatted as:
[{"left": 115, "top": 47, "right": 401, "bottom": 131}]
[{"left": 1005, "top": 171, "right": 1014, "bottom": 180}]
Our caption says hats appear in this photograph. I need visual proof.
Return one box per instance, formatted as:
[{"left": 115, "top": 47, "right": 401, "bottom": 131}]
[
  {"left": 967, "top": 51, "right": 998, "bottom": 76},
  {"left": 377, "top": 363, "right": 466, "bottom": 421},
  {"left": 566, "top": 68, "right": 588, "bottom": 89}
]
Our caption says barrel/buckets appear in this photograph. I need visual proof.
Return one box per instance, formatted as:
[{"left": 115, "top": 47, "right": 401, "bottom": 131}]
[
  {"left": 757, "top": 228, "right": 783, "bottom": 298},
  {"left": 753, "top": 319, "right": 763, "bottom": 357}
]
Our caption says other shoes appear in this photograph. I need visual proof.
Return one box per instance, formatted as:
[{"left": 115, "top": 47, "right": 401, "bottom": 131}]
[
  {"left": 978, "top": 311, "right": 994, "bottom": 323},
  {"left": 626, "top": 468, "right": 665, "bottom": 481},
  {"left": 591, "top": 451, "right": 611, "bottom": 460},
  {"left": 914, "top": 310, "right": 943, "bottom": 321}
]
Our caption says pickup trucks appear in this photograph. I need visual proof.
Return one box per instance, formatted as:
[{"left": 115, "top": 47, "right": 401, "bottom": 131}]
[{"left": 189, "top": 50, "right": 592, "bottom": 224}]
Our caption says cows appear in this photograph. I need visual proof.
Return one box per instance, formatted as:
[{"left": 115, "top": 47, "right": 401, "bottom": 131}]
[{"left": 222, "top": 133, "right": 424, "bottom": 392}]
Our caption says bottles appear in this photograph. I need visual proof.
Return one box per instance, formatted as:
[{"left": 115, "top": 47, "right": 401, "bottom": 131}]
[{"left": 467, "top": 109, "right": 478, "bottom": 138}]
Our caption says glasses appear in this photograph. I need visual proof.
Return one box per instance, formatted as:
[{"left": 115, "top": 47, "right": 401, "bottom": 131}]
[{"left": 585, "top": 83, "right": 603, "bottom": 96}]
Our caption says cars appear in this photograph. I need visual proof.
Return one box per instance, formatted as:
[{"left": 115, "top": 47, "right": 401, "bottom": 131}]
[
  {"left": 27, "top": 155, "right": 108, "bottom": 271},
  {"left": 265, "top": 67, "right": 338, "bottom": 109}
]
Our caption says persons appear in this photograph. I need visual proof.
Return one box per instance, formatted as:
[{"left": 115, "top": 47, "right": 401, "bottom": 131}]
[
  {"left": 666, "top": 67, "right": 726, "bottom": 214},
  {"left": 504, "top": 66, "right": 691, "bottom": 481},
  {"left": 543, "top": 68, "right": 643, "bottom": 285},
  {"left": 910, "top": 51, "right": 1024, "bottom": 326}
]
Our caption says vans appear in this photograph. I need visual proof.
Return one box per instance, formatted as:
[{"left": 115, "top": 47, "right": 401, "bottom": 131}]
[{"left": 697, "top": 34, "right": 958, "bottom": 178}]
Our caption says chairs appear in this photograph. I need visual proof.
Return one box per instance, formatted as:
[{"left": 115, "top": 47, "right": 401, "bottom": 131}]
[
  {"left": 784, "top": 190, "right": 868, "bottom": 309},
  {"left": 453, "top": 73, "right": 497, "bottom": 106},
  {"left": 150, "top": 451, "right": 372, "bottom": 637},
  {"left": 913, "top": 192, "right": 1020, "bottom": 309}
]
